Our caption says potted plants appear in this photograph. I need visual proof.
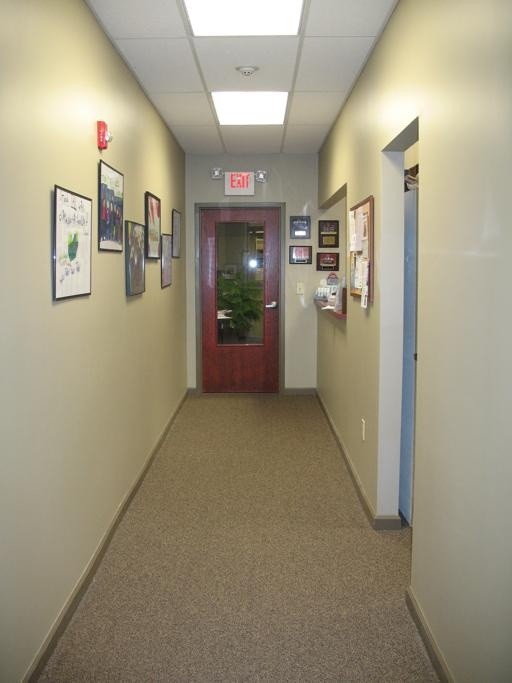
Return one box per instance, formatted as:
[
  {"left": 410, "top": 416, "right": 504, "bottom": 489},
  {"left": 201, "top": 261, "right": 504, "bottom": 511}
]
[{"left": 216, "top": 271, "right": 264, "bottom": 340}]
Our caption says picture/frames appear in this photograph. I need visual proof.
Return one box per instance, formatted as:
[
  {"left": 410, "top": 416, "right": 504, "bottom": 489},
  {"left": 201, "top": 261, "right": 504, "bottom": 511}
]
[
  {"left": 50, "top": 183, "right": 93, "bottom": 302},
  {"left": 158, "top": 232, "right": 174, "bottom": 288},
  {"left": 315, "top": 251, "right": 339, "bottom": 272},
  {"left": 96, "top": 158, "right": 124, "bottom": 254},
  {"left": 142, "top": 189, "right": 162, "bottom": 261},
  {"left": 170, "top": 206, "right": 181, "bottom": 257},
  {"left": 122, "top": 218, "right": 148, "bottom": 298},
  {"left": 289, "top": 214, "right": 311, "bottom": 238},
  {"left": 318, "top": 218, "right": 340, "bottom": 248},
  {"left": 289, "top": 243, "right": 312, "bottom": 264}
]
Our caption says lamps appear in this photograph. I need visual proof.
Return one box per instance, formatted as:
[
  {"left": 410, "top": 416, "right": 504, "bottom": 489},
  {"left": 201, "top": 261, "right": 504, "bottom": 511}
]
[{"left": 210, "top": 167, "right": 268, "bottom": 184}]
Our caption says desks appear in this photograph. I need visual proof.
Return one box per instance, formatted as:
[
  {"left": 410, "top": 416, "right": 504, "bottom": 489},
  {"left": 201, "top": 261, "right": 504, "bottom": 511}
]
[{"left": 216, "top": 308, "right": 233, "bottom": 343}]
